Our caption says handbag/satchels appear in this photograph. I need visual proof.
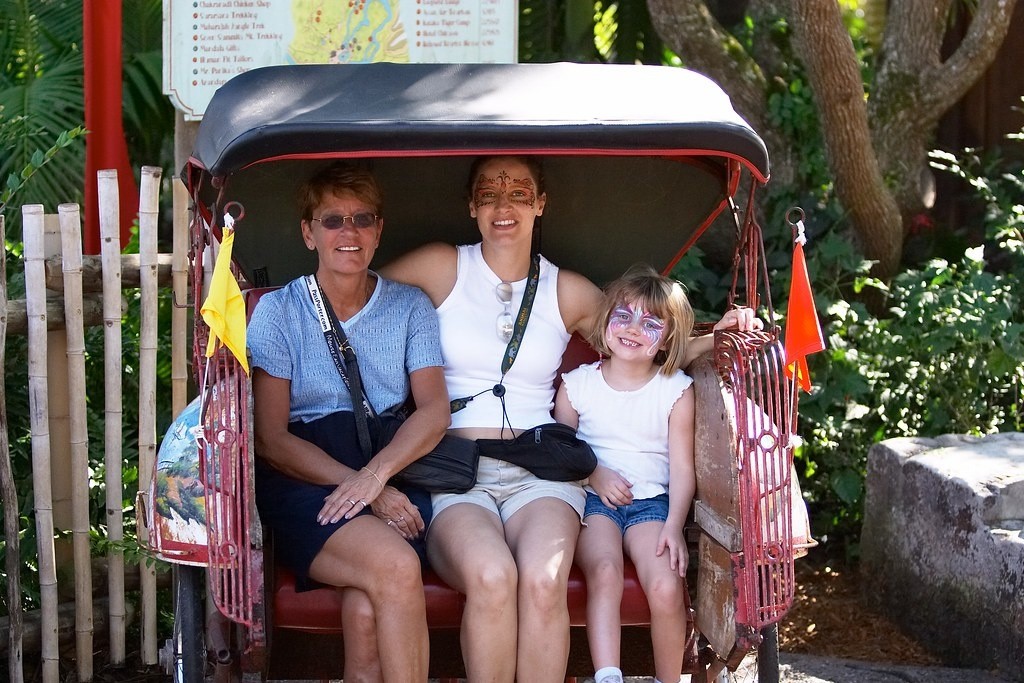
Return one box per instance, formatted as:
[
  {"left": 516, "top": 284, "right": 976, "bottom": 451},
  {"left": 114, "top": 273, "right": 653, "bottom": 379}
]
[
  {"left": 377, "top": 418, "right": 479, "bottom": 493},
  {"left": 475, "top": 422, "right": 598, "bottom": 482}
]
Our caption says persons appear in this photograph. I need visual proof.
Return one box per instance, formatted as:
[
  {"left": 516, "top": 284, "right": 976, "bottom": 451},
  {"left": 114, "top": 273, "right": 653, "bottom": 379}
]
[
  {"left": 555, "top": 272, "right": 696, "bottom": 683},
  {"left": 377, "top": 155, "right": 763, "bottom": 682},
  {"left": 246, "top": 168, "right": 452, "bottom": 683}
]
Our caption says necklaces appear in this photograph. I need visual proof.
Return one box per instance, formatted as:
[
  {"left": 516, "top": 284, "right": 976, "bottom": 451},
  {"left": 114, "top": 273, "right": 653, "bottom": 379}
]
[{"left": 316, "top": 274, "right": 369, "bottom": 351}]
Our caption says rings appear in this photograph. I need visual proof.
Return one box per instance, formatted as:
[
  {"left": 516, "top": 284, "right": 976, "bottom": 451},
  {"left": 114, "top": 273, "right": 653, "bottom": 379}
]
[
  {"left": 395, "top": 517, "right": 403, "bottom": 522},
  {"left": 387, "top": 521, "right": 392, "bottom": 525},
  {"left": 347, "top": 498, "right": 355, "bottom": 506},
  {"left": 360, "top": 500, "right": 366, "bottom": 507}
]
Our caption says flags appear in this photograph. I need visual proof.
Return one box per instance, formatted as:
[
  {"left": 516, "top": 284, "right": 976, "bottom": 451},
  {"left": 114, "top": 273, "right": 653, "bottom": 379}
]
[
  {"left": 200, "top": 225, "right": 250, "bottom": 379},
  {"left": 784, "top": 242, "right": 822, "bottom": 395},
  {"left": 85, "top": 1, "right": 141, "bottom": 253}
]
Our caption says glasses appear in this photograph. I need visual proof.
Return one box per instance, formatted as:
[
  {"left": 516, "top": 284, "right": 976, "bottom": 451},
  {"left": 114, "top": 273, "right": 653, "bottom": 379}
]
[{"left": 310, "top": 213, "right": 380, "bottom": 229}]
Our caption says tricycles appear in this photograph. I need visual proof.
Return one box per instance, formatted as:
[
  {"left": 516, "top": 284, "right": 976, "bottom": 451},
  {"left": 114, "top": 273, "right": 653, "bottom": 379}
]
[{"left": 152, "top": 61, "right": 793, "bottom": 681}]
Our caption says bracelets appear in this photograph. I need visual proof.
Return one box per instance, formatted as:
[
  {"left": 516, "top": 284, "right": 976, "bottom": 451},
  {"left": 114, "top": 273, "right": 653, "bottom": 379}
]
[{"left": 363, "top": 467, "right": 384, "bottom": 487}]
